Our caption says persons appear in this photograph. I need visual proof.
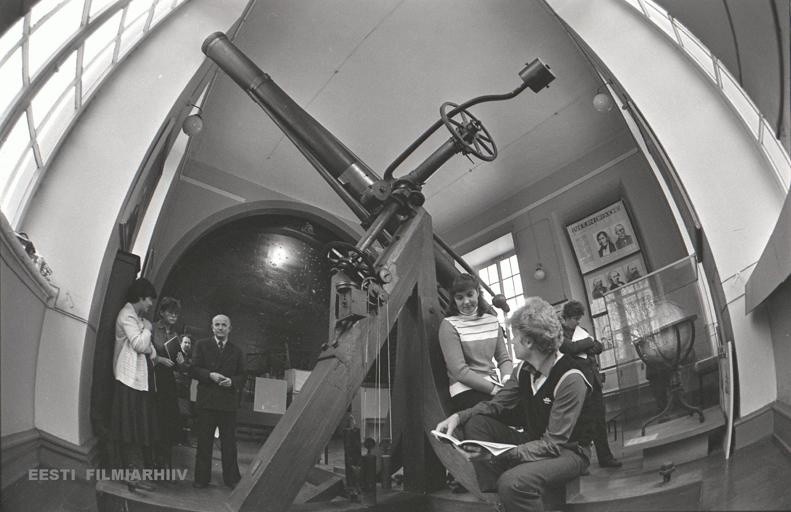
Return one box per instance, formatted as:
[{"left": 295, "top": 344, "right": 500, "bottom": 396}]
[
  {"left": 191, "top": 315, "right": 244, "bottom": 489},
  {"left": 435, "top": 296, "right": 593, "bottom": 509},
  {"left": 556, "top": 301, "right": 621, "bottom": 466},
  {"left": 112, "top": 276, "right": 157, "bottom": 479},
  {"left": 612, "top": 223, "right": 636, "bottom": 248},
  {"left": 437, "top": 275, "right": 513, "bottom": 410},
  {"left": 177, "top": 334, "right": 192, "bottom": 374},
  {"left": 598, "top": 231, "right": 614, "bottom": 256},
  {"left": 152, "top": 295, "right": 184, "bottom": 459}
]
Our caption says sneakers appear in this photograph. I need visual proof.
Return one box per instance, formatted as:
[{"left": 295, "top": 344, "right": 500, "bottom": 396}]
[{"left": 599, "top": 457, "right": 622, "bottom": 467}]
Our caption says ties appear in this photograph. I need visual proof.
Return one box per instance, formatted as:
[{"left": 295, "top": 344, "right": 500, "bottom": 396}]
[{"left": 218, "top": 341, "right": 225, "bottom": 351}]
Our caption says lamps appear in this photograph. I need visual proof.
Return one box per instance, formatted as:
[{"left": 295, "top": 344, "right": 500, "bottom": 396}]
[
  {"left": 533, "top": 263, "right": 546, "bottom": 281},
  {"left": 590, "top": 85, "right": 613, "bottom": 113},
  {"left": 180, "top": 103, "right": 205, "bottom": 138}
]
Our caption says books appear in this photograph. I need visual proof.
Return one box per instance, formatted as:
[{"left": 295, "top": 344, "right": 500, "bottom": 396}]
[{"left": 429, "top": 425, "right": 518, "bottom": 460}]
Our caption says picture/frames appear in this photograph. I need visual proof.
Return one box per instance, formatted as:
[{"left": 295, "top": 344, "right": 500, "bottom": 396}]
[
  {"left": 590, "top": 295, "right": 668, "bottom": 375},
  {"left": 116, "top": 114, "right": 177, "bottom": 256},
  {"left": 560, "top": 197, "right": 643, "bottom": 276},
  {"left": 579, "top": 248, "right": 656, "bottom": 317}
]
